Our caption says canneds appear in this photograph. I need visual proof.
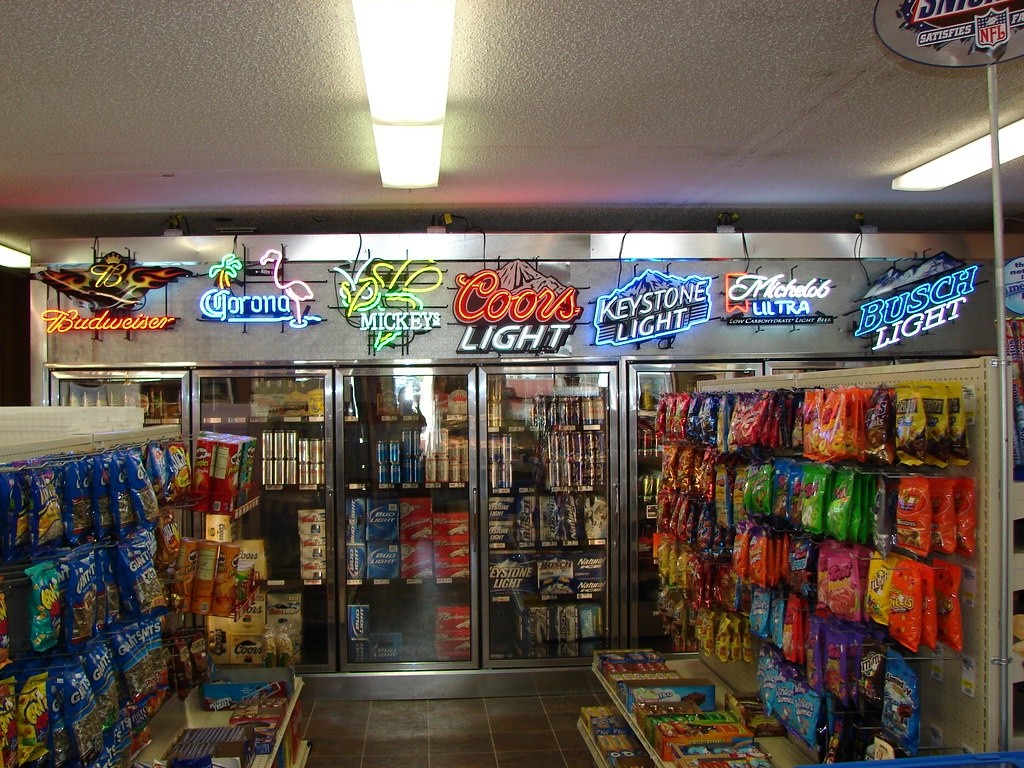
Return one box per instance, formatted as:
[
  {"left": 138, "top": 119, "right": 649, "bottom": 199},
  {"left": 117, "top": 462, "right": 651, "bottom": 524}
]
[
  {"left": 487, "top": 376, "right": 605, "bottom": 489},
  {"left": 637, "top": 430, "right": 663, "bottom": 457},
  {"left": 638, "top": 475, "right": 662, "bottom": 496},
  {"left": 261, "top": 430, "right": 324, "bottom": 485},
  {"left": 378, "top": 427, "right": 469, "bottom": 483}
]
